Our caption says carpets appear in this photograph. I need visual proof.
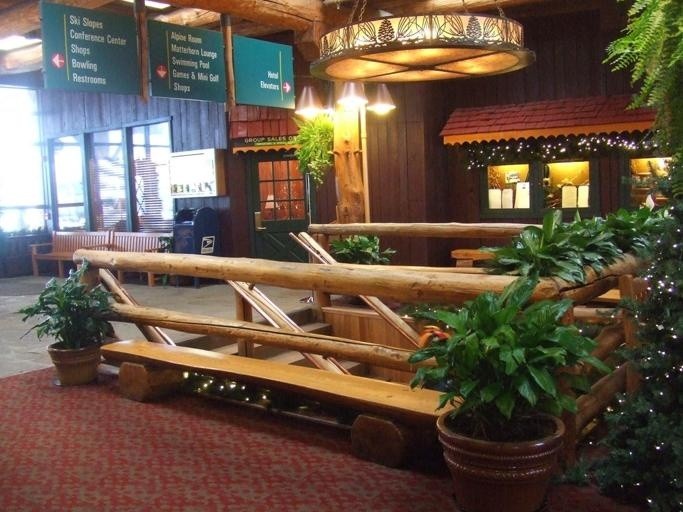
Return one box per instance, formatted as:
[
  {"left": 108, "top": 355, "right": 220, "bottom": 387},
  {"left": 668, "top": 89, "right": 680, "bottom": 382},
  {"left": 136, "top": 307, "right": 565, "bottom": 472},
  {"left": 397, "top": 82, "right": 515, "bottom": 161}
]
[{"left": 0, "top": 367, "right": 643, "bottom": 512}]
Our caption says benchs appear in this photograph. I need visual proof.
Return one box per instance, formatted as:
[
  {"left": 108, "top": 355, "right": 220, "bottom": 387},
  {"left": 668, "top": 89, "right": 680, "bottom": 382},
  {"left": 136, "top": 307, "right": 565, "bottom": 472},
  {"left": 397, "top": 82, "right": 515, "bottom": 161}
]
[
  {"left": 87, "top": 231, "right": 174, "bottom": 287},
  {"left": 451, "top": 249, "right": 496, "bottom": 267},
  {"left": 102, "top": 339, "right": 462, "bottom": 466},
  {"left": 31, "top": 230, "right": 110, "bottom": 276}
]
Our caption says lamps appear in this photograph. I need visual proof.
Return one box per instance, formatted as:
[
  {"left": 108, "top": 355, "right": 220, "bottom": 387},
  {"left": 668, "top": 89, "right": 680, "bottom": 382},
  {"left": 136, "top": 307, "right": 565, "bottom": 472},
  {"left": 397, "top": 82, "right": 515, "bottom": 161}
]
[
  {"left": 310, "top": 1, "right": 538, "bottom": 82},
  {"left": 336, "top": 81, "right": 368, "bottom": 109},
  {"left": 292, "top": 76, "right": 328, "bottom": 116},
  {"left": 368, "top": 83, "right": 397, "bottom": 114},
  {"left": 265, "top": 195, "right": 280, "bottom": 218}
]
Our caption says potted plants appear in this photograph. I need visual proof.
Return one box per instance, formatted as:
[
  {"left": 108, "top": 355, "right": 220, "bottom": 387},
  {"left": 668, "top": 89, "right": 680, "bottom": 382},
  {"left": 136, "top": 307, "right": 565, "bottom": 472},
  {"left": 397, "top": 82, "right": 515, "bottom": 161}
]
[
  {"left": 18, "top": 262, "right": 119, "bottom": 386},
  {"left": 328, "top": 233, "right": 395, "bottom": 304},
  {"left": 411, "top": 272, "right": 612, "bottom": 512}
]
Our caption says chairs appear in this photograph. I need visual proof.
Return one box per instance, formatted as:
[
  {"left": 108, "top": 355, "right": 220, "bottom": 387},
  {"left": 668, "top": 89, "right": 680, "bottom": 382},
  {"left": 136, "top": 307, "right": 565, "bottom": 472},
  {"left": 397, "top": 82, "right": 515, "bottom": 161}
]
[{"left": 618, "top": 273, "right": 670, "bottom": 400}]
